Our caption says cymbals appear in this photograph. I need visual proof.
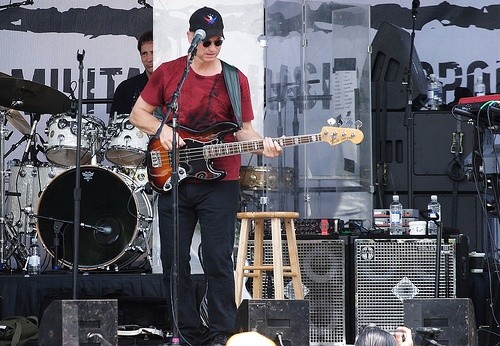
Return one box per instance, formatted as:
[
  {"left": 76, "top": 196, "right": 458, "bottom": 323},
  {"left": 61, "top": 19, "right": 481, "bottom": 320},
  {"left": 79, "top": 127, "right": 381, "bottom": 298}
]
[
  {"left": 0, "top": 105, "right": 33, "bottom": 135},
  {"left": 0, "top": 78, "right": 72, "bottom": 115}
]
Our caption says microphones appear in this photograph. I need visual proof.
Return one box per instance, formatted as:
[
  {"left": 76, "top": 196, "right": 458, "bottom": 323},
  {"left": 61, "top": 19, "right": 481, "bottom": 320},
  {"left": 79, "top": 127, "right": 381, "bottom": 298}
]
[
  {"left": 21, "top": 113, "right": 41, "bottom": 163},
  {"left": 188, "top": 29, "right": 206, "bottom": 54},
  {"left": 412, "top": 0, "right": 420, "bottom": 18},
  {"left": 94, "top": 226, "right": 112, "bottom": 234}
]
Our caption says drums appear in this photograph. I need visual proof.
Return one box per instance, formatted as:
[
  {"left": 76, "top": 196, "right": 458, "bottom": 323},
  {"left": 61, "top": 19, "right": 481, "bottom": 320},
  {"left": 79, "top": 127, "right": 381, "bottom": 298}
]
[
  {"left": 34, "top": 165, "right": 154, "bottom": 273},
  {"left": 239, "top": 166, "right": 295, "bottom": 193},
  {"left": 42, "top": 112, "right": 106, "bottom": 168},
  {"left": 5, "top": 161, "right": 72, "bottom": 238},
  {"left": 104, "top": 114, "right": 150, "bottom": 169}
]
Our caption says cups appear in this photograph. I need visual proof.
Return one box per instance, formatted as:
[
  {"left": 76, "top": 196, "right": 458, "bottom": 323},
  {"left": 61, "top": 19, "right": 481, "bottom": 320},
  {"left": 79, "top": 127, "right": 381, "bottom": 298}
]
[{"left": 408, "top": 221, "right": 427, "bottom": 235}]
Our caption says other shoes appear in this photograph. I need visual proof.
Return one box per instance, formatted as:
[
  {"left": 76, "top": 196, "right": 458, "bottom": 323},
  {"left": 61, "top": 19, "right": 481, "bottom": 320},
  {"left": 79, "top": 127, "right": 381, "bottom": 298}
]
[{"left": 207, "top": 335, "right": 229, "bottom": 346}]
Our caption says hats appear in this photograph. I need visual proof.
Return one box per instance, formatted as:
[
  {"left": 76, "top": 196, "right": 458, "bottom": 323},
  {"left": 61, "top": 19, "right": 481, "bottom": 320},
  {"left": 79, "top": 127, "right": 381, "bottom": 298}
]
[{"left": 188, "top": 6, "right": 225, "bottom": 42}]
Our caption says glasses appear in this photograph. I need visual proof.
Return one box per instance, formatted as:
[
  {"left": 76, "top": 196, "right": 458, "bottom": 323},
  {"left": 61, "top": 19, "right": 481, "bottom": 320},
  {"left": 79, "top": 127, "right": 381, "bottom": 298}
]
[{"left": 202, "top": 40, "right": 223, "bottom": 47}]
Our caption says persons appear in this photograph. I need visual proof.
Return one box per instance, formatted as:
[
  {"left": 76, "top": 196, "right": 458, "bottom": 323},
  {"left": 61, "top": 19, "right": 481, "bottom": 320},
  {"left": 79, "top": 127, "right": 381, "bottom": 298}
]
[
  {"left": 129, "top": 7, "right": 282, "bottom": 346},
  {"left": 109, "top": 31, "right": 153, "bottom": 117},
  {"left": 355, "top": 325, "right": 413, "bottom": 346}
]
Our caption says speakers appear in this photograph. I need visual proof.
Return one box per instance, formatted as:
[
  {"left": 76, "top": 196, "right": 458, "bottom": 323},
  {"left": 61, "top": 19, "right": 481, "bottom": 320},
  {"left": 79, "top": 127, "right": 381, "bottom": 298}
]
[
  {"left": 39, "top": 299, "right": 118, "bottom": 346},
  {"left": 236, "top": 19, "right": 500, "bottom": 346}
]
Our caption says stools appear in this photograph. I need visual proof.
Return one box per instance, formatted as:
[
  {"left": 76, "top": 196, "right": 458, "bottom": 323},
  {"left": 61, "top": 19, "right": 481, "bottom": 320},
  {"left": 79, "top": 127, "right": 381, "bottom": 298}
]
[{"left": 234, "top": 211, "right": 304, "bottom": 309}]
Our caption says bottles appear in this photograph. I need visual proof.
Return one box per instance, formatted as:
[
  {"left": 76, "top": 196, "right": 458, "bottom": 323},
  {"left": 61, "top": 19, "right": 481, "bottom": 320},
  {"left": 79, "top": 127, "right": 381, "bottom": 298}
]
[
  {"left": 428, "top": 195, "right": 441, "bottom": 235},
  {"left": 474, "top": 77, "right": 485, "bottom": 96},
  {"left": 389, "top": 195, "right": 402, "bottom": 236},
  {"left": 170, "top": 338, "right": 181, "bottom": 346},
  {"left": 28, "top": 239, "right": 41, "bottom": 276},
  {"left": 427, "top": 73, "right": 442, "bottom": 110}
]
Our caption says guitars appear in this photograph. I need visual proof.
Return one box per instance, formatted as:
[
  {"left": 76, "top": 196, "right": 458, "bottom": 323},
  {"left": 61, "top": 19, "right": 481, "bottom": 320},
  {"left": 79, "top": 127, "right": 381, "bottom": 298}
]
[{"left": 146, "top": 118, "right": 365, "bottom": 195}]
